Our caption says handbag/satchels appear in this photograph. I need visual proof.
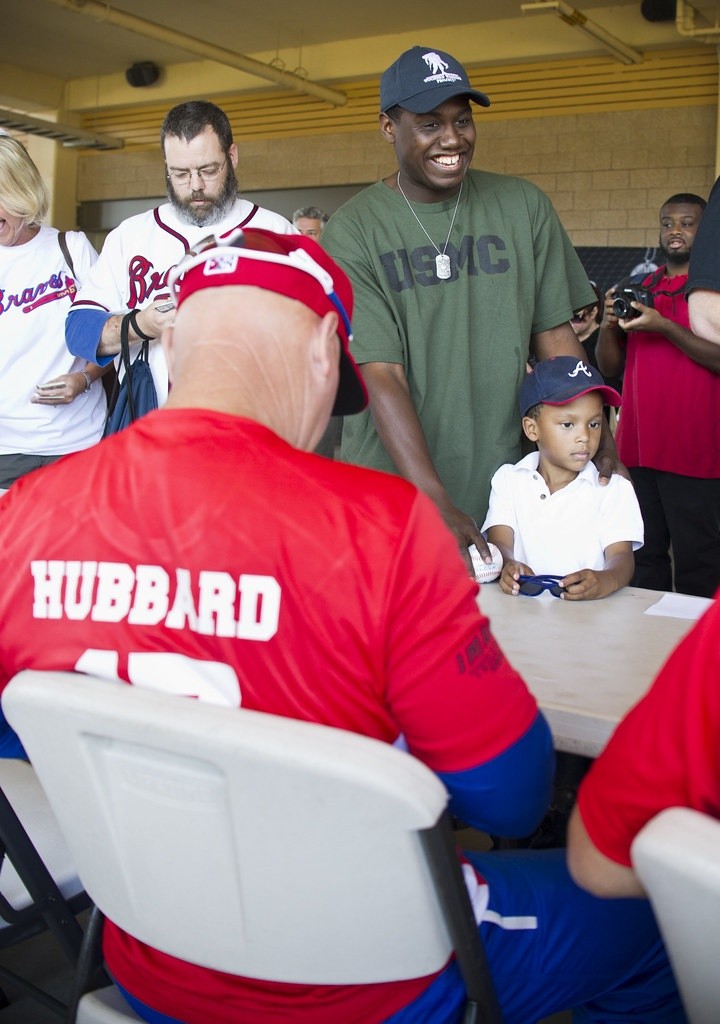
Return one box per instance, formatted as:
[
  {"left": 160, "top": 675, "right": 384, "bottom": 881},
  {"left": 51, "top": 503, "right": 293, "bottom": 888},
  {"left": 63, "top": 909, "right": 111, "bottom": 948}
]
[{"left": 104, "top": 308, "right": 158, "bottom": 448}]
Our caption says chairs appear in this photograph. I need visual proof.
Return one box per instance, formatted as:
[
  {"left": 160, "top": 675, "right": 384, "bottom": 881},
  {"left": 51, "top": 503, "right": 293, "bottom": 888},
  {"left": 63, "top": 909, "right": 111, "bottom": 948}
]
[{"left": 0, "top": 669, "right": 720, "bottom": 1024}]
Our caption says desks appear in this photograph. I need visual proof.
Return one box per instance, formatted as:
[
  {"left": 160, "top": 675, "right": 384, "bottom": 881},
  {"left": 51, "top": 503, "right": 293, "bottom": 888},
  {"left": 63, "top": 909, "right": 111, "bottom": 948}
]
[{"left": 476, "top": 578, "right": 714, "bottom": 850}]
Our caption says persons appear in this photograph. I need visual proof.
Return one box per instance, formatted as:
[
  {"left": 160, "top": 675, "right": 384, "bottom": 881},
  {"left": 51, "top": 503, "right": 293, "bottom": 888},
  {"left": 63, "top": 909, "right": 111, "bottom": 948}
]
[
  {"left": 0, "top": 135, "right": 108, "bottom": 494},
  {"left": 567, "top": 583, "right": 720, "bottom": 897},
  {"left": 293, "top": 206, "right": 330, "bottom": 243},
  {"left": 595, "top": 192, "right": 720, "bottom": 599},
  {"left": 65, "top": 100, "right": 301, "bottom": 407},
  {"left": 569, "top": 281, "right": 622, "bottom": 427},
  {"left": 0, "top": 226, "right": 691, "bottom": 1024},
  {"left": 684, "top": 175, "right": 720, "bottom": 346},
  {"left": 479, "top": 354, "right": 644, "bottom": 600},
  {"left": 315, "top": 45, "right": 632, "bottom": 580}
]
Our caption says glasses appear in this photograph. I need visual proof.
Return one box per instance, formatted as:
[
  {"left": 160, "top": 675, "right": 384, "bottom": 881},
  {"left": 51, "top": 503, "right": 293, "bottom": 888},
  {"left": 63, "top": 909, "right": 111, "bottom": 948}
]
[
  {"left": 514, "top": 575, "right": 568, "bottom": 598},
  {"left": 166, "top": 156, "right": 230, "bottom": 185},
  {"left": 168, "top": 230, "right": 354, "bottom": 340}
]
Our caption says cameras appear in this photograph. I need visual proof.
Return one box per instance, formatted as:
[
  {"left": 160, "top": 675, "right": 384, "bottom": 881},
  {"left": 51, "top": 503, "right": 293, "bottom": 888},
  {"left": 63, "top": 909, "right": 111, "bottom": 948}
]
[{"left": 612, "top": 283, "right": 655, "bottom": 319}]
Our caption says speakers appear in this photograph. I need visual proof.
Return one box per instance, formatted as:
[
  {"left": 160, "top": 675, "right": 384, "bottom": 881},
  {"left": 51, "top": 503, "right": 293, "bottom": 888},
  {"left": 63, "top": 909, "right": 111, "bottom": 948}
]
[
  {"left": 641, "top": 0, "right": 677, "bottom": 22},
  {"left": 126, "top": 61, "right": 158, "bottom": 88}
]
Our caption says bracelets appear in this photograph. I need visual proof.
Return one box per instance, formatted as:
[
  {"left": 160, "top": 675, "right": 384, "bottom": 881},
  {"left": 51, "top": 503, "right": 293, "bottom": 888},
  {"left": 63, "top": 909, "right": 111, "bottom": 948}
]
[{"left": 81, "top": 370, "right": 92, "bottom": 395}]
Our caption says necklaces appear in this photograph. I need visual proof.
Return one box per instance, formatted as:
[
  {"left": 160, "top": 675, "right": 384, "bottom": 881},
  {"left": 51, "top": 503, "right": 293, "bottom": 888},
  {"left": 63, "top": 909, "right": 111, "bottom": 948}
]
[{"left": 397, "top": 171, "right": 463, "bottom": 279}]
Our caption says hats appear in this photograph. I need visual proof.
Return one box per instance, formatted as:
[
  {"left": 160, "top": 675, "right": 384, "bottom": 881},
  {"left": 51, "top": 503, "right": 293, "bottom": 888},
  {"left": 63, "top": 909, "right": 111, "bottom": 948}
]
[
  {"left": 380, "top": 45, "right": 491, "bottom": 114},
  {"left": 176, "top": 228, "right": 369, "bottom": 417},
  {"left": 518, "top": 355, "right": 622, "bottom": 419}
]
